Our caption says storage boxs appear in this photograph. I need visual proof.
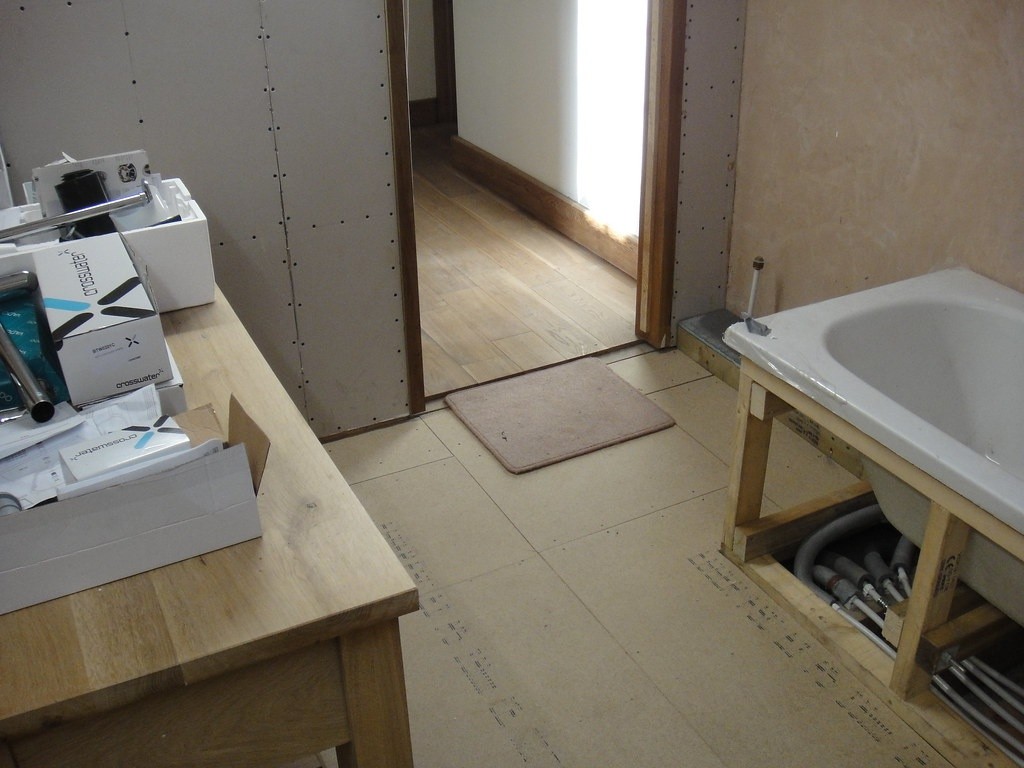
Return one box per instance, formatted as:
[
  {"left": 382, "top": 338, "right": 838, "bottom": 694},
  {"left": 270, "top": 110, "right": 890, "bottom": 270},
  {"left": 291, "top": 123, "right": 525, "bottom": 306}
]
[
  {"left": 0, "top": 176, "right": 216, "bottom": 317},
  {"left": 0, "top": 394, "right": 273, "bottom": 622},
  {"left": 156, "top": 338, "right": 188, "bottom": 421},
  {"left": 36, "top": 230, "right": 171, "bottom": 411}
]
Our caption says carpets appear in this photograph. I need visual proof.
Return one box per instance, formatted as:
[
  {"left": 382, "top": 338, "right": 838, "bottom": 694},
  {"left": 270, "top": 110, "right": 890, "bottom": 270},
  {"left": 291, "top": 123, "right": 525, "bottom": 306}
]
[{"left": 439, "top": 353, "right": 679, "bottom": 473}]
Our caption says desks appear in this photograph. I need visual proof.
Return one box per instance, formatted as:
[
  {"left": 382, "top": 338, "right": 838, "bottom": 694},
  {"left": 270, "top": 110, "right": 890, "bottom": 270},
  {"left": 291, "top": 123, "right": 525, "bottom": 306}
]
[{"left": 0, "top": 279, "right": 424, "bottom": 768}]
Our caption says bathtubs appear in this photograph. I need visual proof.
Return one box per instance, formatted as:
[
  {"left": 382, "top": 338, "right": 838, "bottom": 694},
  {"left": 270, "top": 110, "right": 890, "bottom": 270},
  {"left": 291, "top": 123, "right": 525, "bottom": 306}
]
[{"left": 719, "top": 265, "right": 1023, "bottom": 629}]
